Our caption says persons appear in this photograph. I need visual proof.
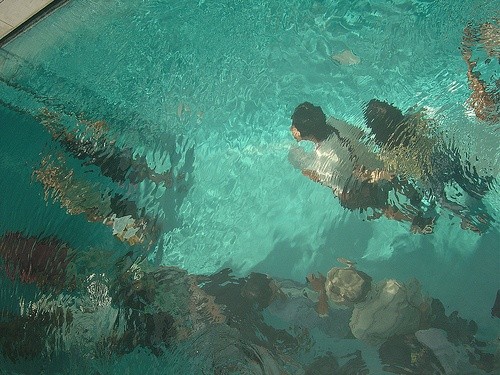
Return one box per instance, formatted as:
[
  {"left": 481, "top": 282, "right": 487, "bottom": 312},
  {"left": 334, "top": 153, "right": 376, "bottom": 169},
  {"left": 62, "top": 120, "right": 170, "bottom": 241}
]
[
  {"left": 1, "top": 232, "right": 102, "bottom": 289},
  {"left": 303, "top": 349, "right": 370, "bottom": 374},
  {"left": 461, "top": 16, "right": 500, "bottom": 126},
  {"left": 307, "top": 266, "right": 479, "bottom": 347},
  {"left": 236, "top": 272, "right": 357, "bottom": 338},
  {"left": 291, "top": 99, "right": 434, "bottom": 234},
  {"left": 353, "top": 100, "right": 488, "bottom": 233},
  {"left": 1, "top": 293, "right": 139, "bottom": 362},
  {"left": 112, "top": 257, "right": 296, "bottom": 375},
  {"left": 378, "top": 329, "right": 499, "bottom": 375},
  {"left": 37, "top": 108, "right": 173, "bottom": 188},
  {"left": 31, "top": 163, "right": 157, "bottom": 243}
]
